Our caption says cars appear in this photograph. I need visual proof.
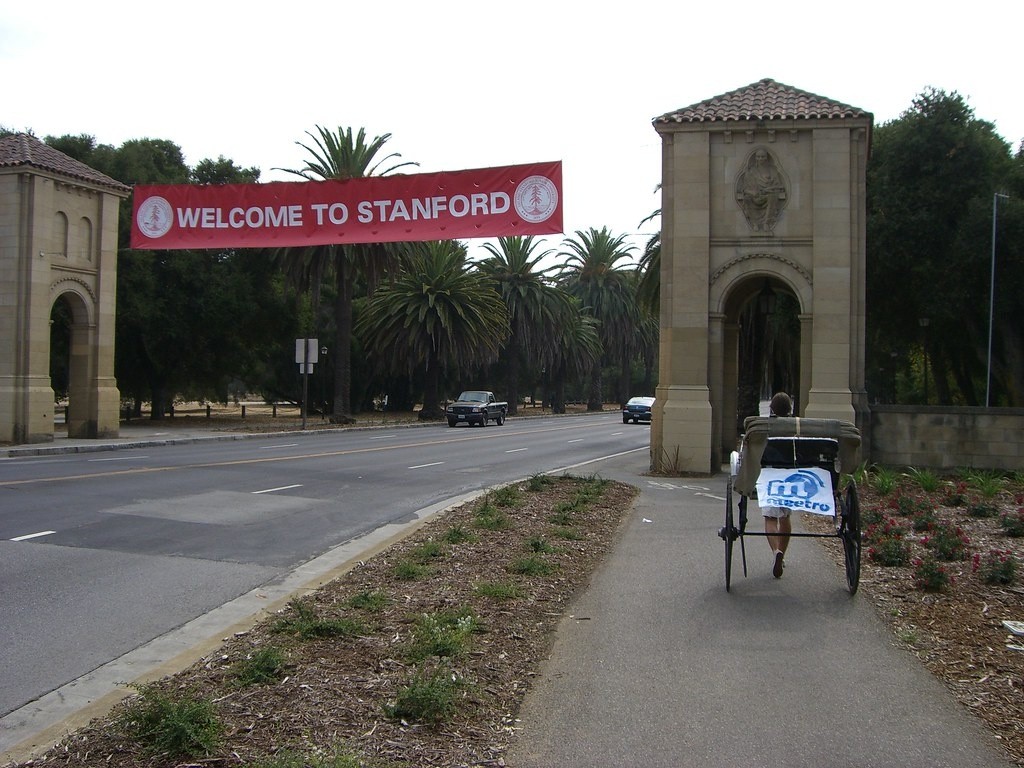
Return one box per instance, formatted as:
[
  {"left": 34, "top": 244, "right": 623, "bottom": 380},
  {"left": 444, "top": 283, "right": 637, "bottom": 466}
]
[{"left": 623, "top": 397, "right": 655, "bottom": 424}]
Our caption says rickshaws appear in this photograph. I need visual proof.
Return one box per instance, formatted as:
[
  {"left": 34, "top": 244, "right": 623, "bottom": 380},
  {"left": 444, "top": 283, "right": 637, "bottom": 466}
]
[{"left": 717, "top": 416, "right": 863, "bottom": 595}]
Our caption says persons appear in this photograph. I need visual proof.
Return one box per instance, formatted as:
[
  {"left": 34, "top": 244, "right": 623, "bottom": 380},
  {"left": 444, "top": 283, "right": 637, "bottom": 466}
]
[
  {"left": 762, "top": 393, "right": 791, "bottom": 578},
  {"left": 744, "top": 149, "right": 780, "bottom": 232}
]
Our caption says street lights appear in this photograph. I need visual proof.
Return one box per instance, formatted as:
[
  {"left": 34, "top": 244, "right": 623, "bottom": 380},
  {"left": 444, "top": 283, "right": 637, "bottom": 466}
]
[{"left": 318, "top": 346, "right": 330, "bottom": 425}]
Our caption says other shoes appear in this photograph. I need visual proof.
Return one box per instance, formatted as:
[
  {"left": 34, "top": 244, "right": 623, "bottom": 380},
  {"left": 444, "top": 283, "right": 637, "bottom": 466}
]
[{"left": 771, "top": 549, "right": 784, "bottom": 578}]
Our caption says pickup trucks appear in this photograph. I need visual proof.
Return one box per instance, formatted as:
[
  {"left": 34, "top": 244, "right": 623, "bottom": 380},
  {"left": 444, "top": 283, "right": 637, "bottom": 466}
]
[{"left": 446, "top": 391, "right": 507, "bottom": 427}]
